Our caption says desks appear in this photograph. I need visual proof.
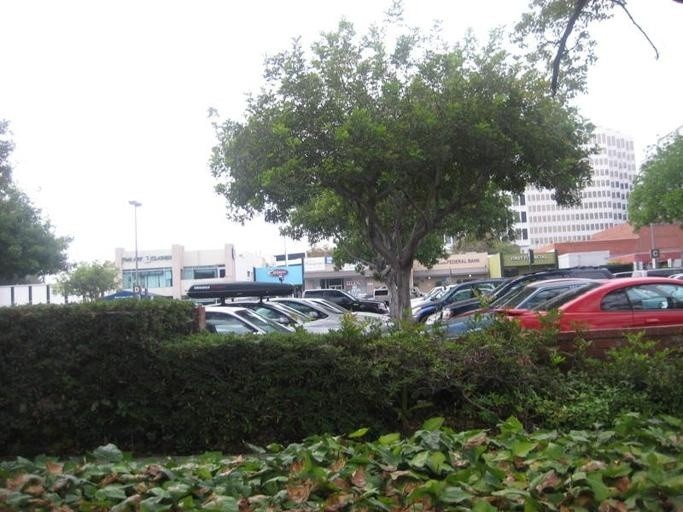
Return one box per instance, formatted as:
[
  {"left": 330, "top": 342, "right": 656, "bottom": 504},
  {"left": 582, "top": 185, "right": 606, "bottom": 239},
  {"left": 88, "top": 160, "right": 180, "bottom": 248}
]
[{"left": 128, "top": 199, "right": 141, "bottom": 286}]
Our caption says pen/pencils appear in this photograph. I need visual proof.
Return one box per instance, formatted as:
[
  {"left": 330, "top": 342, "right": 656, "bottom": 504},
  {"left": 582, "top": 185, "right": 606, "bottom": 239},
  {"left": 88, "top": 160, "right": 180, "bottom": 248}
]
[{"left": 374, "top": 289, "right": 390, "bottom": 305}]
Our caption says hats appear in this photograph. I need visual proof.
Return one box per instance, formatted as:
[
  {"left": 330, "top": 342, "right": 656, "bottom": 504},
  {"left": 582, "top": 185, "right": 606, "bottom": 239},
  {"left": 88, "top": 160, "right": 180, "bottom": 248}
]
[
  {"left": 182, "top": 289, "right": 388, "bottom": 336},
  {"left": 105, "top": 291, "right": 174, "bottom": 301},
  {"left": 409, "top": 269, "right": 683, "bottom": 342}
]
